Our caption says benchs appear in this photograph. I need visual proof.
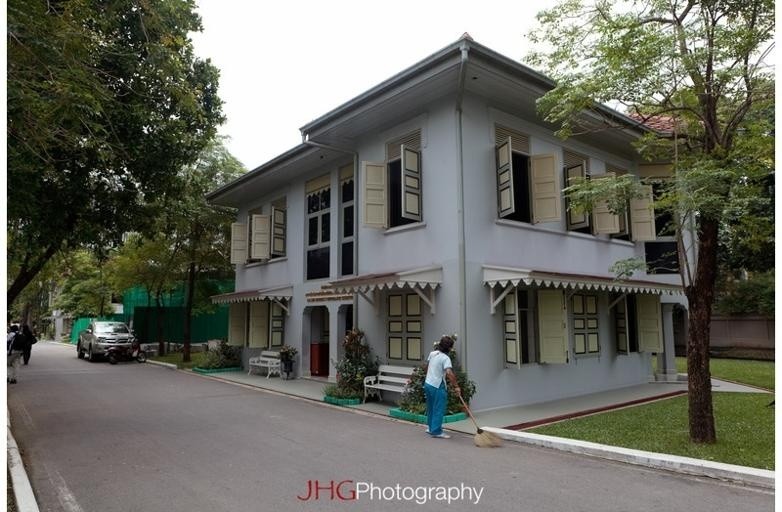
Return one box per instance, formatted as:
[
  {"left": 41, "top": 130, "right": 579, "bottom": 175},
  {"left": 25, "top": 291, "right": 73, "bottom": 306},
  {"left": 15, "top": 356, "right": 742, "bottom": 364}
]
[
  {"left": 362, "top": 364, "right": 419, "bottom": 408},
  {"left": 246, "top": 350, "right": 284, "bottom": 380}
]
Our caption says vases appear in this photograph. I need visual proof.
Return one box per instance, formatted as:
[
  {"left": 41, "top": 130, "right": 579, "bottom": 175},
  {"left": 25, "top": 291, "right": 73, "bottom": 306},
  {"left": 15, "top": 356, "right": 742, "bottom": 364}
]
[{"left": 279, "top": 352, "right": 296, "bottom": 373}]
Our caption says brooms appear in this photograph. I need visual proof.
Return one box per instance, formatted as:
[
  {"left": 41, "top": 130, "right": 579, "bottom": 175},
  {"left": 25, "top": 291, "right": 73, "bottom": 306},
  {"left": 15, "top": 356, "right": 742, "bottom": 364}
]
[{"left": 459, "top": 394, "right": 506, "bottom": 447}]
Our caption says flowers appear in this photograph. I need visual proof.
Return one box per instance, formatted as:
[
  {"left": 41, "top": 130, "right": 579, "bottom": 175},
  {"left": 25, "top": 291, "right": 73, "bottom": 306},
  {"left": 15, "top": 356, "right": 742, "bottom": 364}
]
[{"left": 278, "top": 343, "right": 300, "bottom": 362}]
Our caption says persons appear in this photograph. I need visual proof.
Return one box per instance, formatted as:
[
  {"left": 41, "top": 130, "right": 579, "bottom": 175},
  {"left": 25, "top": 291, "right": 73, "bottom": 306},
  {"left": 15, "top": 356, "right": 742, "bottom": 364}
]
[
  {"left": 8, "top": 325, "right": 24, "bottom": 385},
  {"left": 423, "top": 336, "right": 462, "bottom": 440},
  {"left": 22, "top": 324, "right": 34, "bottom": 365}
]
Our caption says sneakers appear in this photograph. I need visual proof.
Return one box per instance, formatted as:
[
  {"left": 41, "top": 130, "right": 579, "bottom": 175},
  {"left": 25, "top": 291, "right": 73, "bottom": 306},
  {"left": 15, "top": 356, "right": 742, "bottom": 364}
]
[
  {"left": 427, "top": 428, "right": 450, "bottom": 438},
  {"left": 8, "top": 377, "right": 17, "bottom": 384}
]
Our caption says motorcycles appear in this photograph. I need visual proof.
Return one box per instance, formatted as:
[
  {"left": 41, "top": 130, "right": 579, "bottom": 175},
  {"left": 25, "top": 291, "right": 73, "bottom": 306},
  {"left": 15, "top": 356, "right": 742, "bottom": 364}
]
[{"left": 105, "top": 335, "right": 147, "bottom": 365}]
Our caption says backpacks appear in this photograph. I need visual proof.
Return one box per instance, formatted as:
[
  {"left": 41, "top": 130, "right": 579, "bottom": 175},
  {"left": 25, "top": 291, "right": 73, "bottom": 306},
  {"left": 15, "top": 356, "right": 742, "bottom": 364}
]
[{"left": 11, "top": 330, "right": 25, "bottom": 351}]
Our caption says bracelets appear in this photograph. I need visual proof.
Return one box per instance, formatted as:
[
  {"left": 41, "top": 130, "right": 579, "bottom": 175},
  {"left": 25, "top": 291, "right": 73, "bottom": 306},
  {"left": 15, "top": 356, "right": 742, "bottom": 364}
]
[{"left": 453, "top": 383, "right": 458, "bottom": 388}]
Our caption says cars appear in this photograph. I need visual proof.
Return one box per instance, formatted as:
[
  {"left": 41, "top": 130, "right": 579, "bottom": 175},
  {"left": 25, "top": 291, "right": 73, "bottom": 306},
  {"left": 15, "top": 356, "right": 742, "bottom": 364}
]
[{"left": 76, "top": 321, "right": 140, "bottom": 363}]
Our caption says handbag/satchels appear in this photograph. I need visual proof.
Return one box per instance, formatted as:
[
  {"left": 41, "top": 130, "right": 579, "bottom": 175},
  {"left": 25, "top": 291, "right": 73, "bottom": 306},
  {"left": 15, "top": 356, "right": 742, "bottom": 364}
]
[{"left": 31, "top": 335, "right": 37, "bottom": 344}]
